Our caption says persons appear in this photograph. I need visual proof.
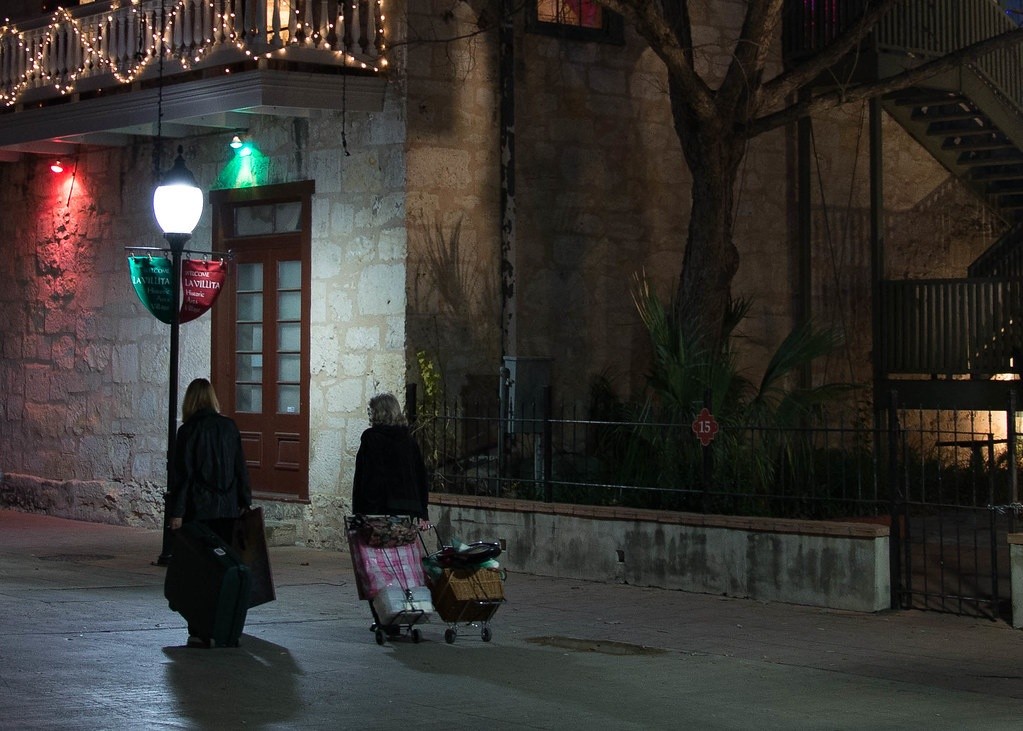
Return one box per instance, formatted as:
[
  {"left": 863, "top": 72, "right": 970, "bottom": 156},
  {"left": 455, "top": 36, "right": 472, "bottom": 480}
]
[
  {"left": 164, "top": 378, "right": 252, "bottom": 646},
  {"left": 352, "top": 393, "right": 430, "bottom": 634}
]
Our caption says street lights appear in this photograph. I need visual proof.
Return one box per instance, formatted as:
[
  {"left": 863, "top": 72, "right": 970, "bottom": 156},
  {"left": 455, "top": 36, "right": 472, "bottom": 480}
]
[{"left": 153, "top": 145, "right": 204, "bottom": 566}]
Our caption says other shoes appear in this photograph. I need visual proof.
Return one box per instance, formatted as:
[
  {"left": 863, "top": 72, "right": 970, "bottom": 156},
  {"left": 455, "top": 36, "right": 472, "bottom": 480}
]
[{"left": 370, "top": 622, "right": 400, "bottom": 636}]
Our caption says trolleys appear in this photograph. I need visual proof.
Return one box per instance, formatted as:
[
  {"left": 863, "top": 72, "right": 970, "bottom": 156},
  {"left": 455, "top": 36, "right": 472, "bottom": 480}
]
[
  {"left": 345, "top": 514, "right": 425, "bottom": 645},
  {"left": 416, "top": 525, "right": 508, "bottom": 644}
]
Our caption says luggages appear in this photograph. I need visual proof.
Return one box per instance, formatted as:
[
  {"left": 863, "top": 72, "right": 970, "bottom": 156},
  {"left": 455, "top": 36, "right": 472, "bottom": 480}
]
[{"left": 164, "top": 523, "right": 251, "bottom": 648}]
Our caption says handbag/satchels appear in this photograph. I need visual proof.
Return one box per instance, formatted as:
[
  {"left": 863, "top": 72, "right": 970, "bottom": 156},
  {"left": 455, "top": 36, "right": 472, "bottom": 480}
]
[
  {"left": 348, "top": 538, "right": 426, "bottom": 599},
  {"left": 233, "top": 504, "right": 277, "bottom": 608},
  {"left": 348, "top": 513, "right": 418, "bottom": 549}
]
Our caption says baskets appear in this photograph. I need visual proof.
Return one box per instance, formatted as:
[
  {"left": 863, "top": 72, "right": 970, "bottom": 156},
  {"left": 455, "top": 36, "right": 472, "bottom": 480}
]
[{"left": 429, "top": 567, "right": 503, "bottom": 622}]
